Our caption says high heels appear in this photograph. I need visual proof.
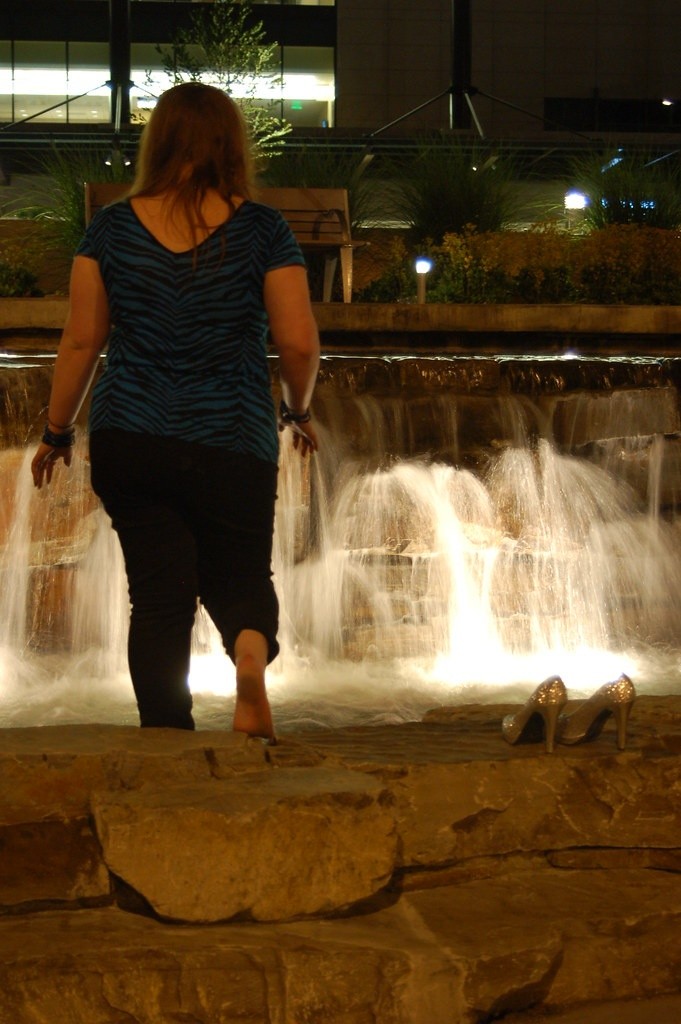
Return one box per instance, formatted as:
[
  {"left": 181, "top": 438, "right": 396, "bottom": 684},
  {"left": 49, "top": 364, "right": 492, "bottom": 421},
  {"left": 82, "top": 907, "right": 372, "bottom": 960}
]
[
  {"left": 501, "top": 675, "right": 568, "bottom": 754},
  {"left": 543, "top": 673, "right": 637, "bottom": 751}
]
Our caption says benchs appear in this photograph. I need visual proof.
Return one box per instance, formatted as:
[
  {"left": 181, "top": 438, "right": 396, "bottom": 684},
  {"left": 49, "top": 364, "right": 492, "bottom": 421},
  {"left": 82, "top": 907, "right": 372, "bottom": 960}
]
[{"left": 83, "top": 181, "right": 368, "bottom": 304}]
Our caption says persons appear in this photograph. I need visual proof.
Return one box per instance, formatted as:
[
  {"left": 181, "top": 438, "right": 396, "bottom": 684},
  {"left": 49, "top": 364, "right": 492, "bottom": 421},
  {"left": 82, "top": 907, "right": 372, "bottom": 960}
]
[{"left": 30, "top": 82, "right": 319, "bottom": 736}]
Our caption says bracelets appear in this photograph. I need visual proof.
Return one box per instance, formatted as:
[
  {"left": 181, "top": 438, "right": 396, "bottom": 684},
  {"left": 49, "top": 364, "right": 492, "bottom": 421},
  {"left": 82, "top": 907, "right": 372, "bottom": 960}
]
[
  {"left": 278, "top": 401, "right": 313, "bottom": 424},
  {"left": 42, "top": 418, "right": 76, "bottom": 447}
]
